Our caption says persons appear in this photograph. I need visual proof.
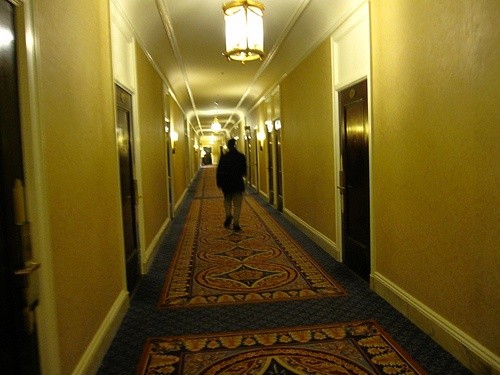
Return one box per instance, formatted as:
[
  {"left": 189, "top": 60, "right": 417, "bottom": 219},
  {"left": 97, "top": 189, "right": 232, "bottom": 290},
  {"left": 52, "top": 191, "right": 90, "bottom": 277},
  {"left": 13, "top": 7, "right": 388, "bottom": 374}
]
[{"left": 216, "top": 138, "right": 247, "bottom": 229}]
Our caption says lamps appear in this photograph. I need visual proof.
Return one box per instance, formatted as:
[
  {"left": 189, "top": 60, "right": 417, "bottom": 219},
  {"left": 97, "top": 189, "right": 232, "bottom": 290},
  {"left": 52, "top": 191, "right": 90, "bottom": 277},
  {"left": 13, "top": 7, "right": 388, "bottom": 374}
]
[{"left": 222, "top": 0, "right": 267, "bottom": 65}]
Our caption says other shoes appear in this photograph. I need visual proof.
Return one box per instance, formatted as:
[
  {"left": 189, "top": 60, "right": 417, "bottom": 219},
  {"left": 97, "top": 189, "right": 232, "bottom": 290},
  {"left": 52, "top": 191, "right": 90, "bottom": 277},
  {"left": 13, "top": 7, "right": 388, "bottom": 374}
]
[
  {"left": 233, "top": 224, "right": 240, "bottom": 230},
  {"left": 225, "top": 216, "right": 233, "bottom": 228}
]
